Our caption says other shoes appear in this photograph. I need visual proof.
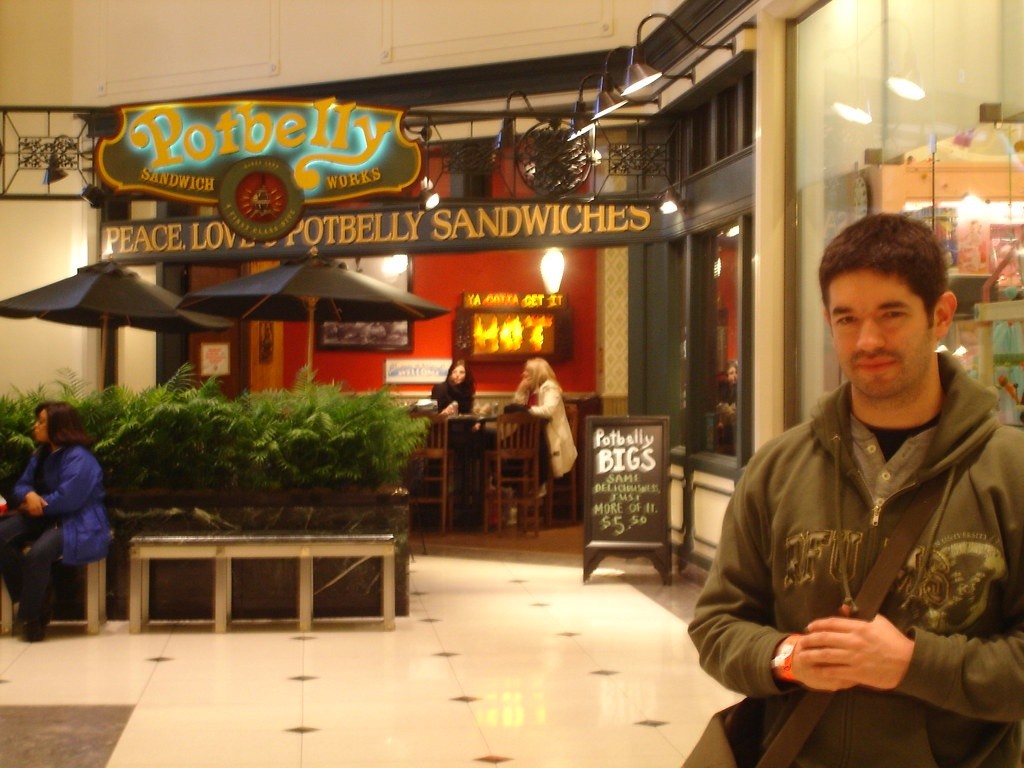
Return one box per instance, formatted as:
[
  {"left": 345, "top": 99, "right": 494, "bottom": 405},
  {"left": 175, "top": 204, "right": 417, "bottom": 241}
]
[{"left": 24, "top": 615, "right": 43, "bottom": 642}]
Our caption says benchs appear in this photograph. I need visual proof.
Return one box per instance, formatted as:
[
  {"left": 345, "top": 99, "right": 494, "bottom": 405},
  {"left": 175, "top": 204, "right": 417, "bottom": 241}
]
[
  {"left": 0, "top": 530, "right": 114, "bottom": 634},
  {"left": 130, "top": 530, "right": 395, "bottom": 633}
]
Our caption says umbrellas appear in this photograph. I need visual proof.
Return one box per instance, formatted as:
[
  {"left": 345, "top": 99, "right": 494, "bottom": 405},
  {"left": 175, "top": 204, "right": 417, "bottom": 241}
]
[
  {"left": 0, "top": 253, "right": 234, "bottom": 403},
  {"left": 177, "top": 258, "right": 451, "bottom": 385}
]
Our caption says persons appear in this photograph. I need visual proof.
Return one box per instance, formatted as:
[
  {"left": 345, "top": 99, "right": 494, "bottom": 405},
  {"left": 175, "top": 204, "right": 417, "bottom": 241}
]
[
  {"left": 513, "top": 358, "right": 578, "bottom": 500},
  {"left": 678, "top": 211, "right": 1024, "bottom": 768},
  {"left": 0, "top": 401, "right": 115, "bottom": 641},
  {"left": 432, "top": 357, "right": 479, "bottom": 459},
  {"left": 717, "top": 360, "right": 737, "bottom": 454},
  {"left": 324, "top": 320, "right": 387, "bottom": 342}
]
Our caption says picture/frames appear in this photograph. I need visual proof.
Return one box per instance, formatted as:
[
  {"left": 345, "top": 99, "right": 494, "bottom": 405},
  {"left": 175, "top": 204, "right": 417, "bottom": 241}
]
[
  {"left": 313, "top": 254, "right": 414, "bottom": 352},
  {"left": 201, "top": 342, "right": 231, "bottom": 377}
]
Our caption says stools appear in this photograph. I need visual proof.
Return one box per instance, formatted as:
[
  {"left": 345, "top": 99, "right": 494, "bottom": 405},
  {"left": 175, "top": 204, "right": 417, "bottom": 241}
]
[
  {"left": 484, "top": 412, "right": 541, "bottom": 535},
  {"left": 406, "top": 412, "right": 455, "bottom": 534},
  {"left": 548, "top": 405, "right": 579, "bottom": 528}
]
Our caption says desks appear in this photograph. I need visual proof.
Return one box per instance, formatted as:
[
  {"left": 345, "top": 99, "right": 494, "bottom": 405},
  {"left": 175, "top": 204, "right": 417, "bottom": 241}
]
[{"left": 441, "top": 416, "right": 498, "bottom": 449}]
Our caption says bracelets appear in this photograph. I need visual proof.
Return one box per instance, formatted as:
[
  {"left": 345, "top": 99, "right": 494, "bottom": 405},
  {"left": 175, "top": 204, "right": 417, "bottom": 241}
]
[{"left": 770, "top": 635, "right": 801, "bottom": 681}]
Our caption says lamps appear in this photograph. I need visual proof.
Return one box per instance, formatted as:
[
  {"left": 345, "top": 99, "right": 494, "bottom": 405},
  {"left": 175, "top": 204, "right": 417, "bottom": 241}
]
[
  {"left": 419, "top": 188, "right": 440, "bottom": 211},
  {"left": 620, "top": 13, "right": 734, "bottom": 97},
  {"left": 568, "top": 72, "right": 663, "bottom": 141},
  {"left": 43, "top": 134, "right": 95, "bottom": 185},
  {"left": 496, "top": 91, "right": 562, "bottom": 148},
  {"left": 591, "top": 45, "right": 696, "bottom": 121},
  {"left": 660, "top": 190, "right": 687, "bottom": 214}
]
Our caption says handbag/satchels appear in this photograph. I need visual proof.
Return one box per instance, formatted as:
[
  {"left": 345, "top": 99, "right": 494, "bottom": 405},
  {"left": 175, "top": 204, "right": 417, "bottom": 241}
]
[{"left": 682, "top": 700, "right": 754, "bottom": 768}]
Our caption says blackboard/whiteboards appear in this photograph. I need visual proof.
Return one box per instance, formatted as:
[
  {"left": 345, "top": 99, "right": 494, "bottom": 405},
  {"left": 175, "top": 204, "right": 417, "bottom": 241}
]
[{"left": 583, "top": 416, "right": 672, "bottom": 560}]
